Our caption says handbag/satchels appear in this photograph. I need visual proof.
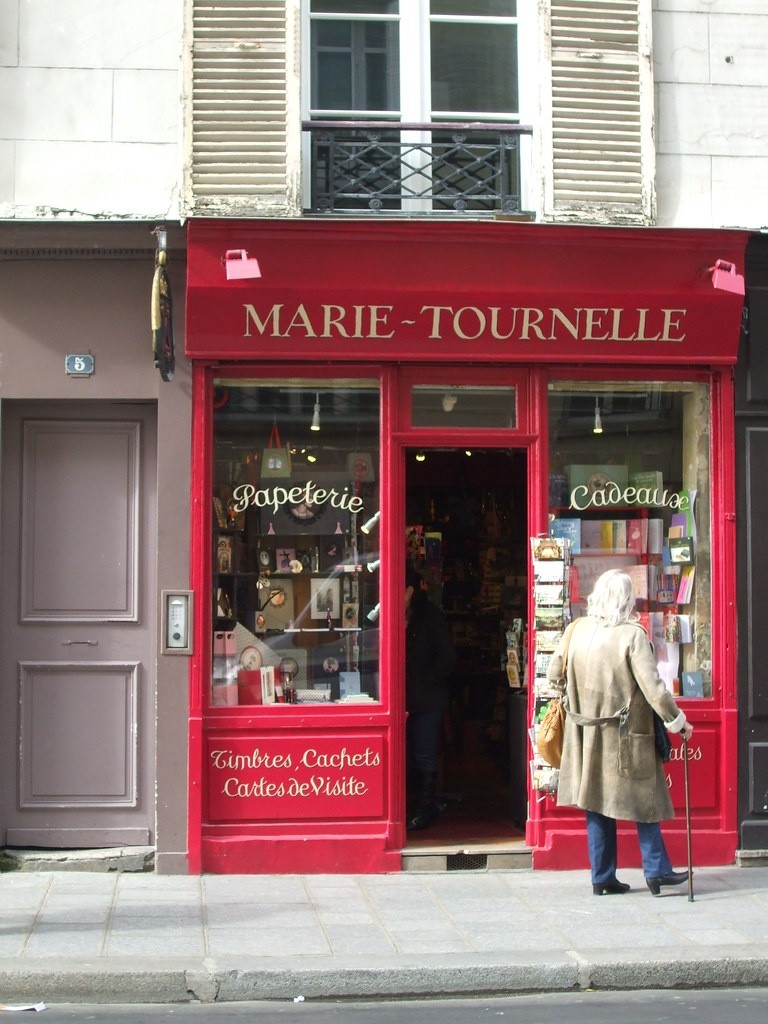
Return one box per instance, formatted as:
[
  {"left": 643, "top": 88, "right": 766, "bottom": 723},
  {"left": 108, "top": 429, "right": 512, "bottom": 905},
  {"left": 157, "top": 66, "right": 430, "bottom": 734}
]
[{"left": 536, "top": 701, "right": 566, "bottom": 769}]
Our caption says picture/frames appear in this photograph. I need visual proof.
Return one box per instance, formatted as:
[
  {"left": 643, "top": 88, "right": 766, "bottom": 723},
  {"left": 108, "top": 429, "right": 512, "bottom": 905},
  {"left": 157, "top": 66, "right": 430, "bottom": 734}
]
[{"left": 310, "top": 578, "right": 340, "bottom": 620}]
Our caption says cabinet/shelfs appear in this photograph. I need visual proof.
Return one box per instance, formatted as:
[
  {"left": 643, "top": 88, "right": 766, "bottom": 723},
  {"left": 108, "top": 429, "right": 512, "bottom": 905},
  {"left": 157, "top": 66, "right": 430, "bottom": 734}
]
[
  {"left": 211, "top": 452, "right": 362, "bottom": 708},
  {"left": 549, "top": 505, "right": 649, "bottom": 695}
]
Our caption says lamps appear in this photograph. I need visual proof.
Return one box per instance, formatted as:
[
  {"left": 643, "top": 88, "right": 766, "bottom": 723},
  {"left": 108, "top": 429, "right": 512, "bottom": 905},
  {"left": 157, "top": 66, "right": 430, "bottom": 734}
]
[
  {"left": 310, "top": 392, "right": 320, "bottom": 431},
  {"left": 593, "top": 396, "right": 603, "bottom": 433}
]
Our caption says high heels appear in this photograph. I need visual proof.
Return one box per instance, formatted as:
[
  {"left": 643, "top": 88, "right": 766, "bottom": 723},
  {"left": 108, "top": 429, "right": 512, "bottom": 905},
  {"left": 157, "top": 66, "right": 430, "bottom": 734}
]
[
  {"left": 592, "top": 875, "right": 630, "bottom": 895},
  {"left": 646, "top": 870, "right": 694, "bottom": 895}
]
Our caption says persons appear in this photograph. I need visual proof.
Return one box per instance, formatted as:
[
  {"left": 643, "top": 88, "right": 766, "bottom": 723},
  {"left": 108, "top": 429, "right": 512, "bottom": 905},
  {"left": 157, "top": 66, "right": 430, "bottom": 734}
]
[
  {"left": 545, "top": 568, "right": 694, "bottom": 895},
  {"left": 405, "top": 566, "right": 455, "bottom": 832}
]
